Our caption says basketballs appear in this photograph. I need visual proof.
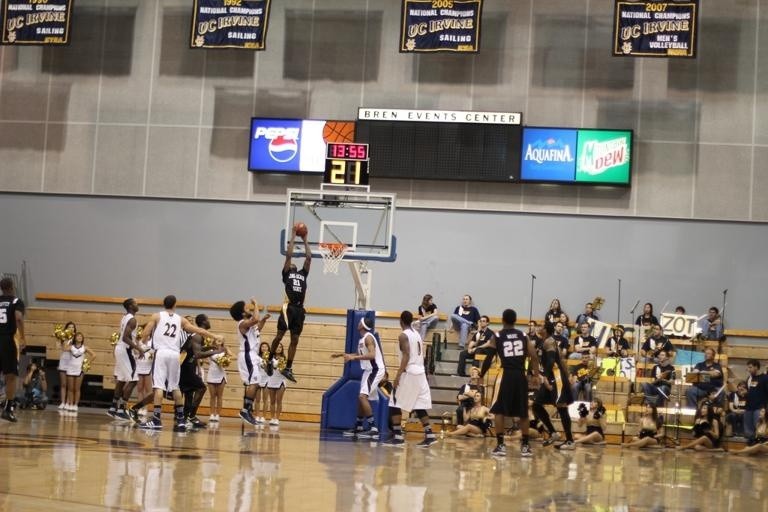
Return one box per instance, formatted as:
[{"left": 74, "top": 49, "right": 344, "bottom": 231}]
[{"left": 295, "top": 222, "right": 307, "bottom": 236}]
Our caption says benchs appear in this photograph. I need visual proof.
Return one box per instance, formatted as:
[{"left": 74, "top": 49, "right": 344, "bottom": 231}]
[{"left": 13, "top": 302, "right": 732, "bottom": 446}]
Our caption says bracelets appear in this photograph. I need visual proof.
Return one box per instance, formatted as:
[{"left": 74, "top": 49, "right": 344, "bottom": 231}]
[{"left": 533, "top": 374, "right": 538, "bottom": 377}]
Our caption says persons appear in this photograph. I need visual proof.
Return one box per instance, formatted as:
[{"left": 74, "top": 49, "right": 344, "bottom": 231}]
[
  {"left": 643, "top": 318, "right": 654, "bottom": 340},
  {"left": 641, "top": 323, "right": 676, "bottom": 359},
  {"left": 686, "top": 348, "right": 725, "bottom": 410},
  {"left": 604, "top": 324, "right": 630, "bottom": 357},
  {"left": 106, "top": 294, "right": 238, "bottom": 433},
  {"left": 230, "top": 296, "right": 271, "bottom": 425},
  {"left": 269, "top": 344, "right": 288, "bottom": 425},
  {"left": 265, "top": 224, "right": 312, "bottom": 384},
  {"left": 254, "top": 342, "right": 270, "bottom": 423},
  {"left": 378, "top": 372, "right": 393, "bottom": 398},
  {"left": 456, "top": 367, "right": 485, "bottom": 426},
  {"left": 63, "top": 331, "right": 96, "bottom": 412},
  {"left": 675, "top": 401, "right": 725, "bottom": 451},
  {"left": 621, "top": 403, "right": 665, "bottom": 449},
  {"left": 526, "top": 298, "right": 608, "bottom": 445},
  {"left": 330, "top": 317, "right": 385, "bottom": 437},
  {"left": 668, "top": 306, "right": 691, "bottom": 339},
  {"left": 695, "top": 307, "right": 726, "bottom": 344},
  {"left": 383, "top": 310, "right": 439, "bottom": 446},
  {"left": 23, "top": 358, "right": 47, "bottom": 410},
  {"left": 439, "top": 392, "right": 495, "bottom": 438},
  {"left": 451, "top": 315, "right": 497, "bottom": 378},
  {"left": 634, "top": 303, "right": 658, "bottom": 325},
  {"left": 411, "top": 294, "right": 439, "bottom": 341},
  {"left": 448, "top": 294, "right": 480, "bottom": 350},
  {"left": 58, "top": 321, "right": 76, "bottom": 409},
  {"left": 531, "top": 322, "right": 576, "bottom": 449},
  {"left": 697, "top": 388, "right": 727, "bottom": 439},
  {"left": 2, "top": 276, "right": 27, "bottom": 422},
  {"left": 726, "top": 358, "right": 767, "bottom": 455},
  {"left": 477, "top": 309, "right": 541, "bottom": 457}
]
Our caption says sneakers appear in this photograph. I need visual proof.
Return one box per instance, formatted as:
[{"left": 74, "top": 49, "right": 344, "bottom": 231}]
[
  {"left": 519, "top": 444, "right": 534, "bottom": 457},
  {"left": 342, "top": 427, "right": 380, "bottom": 440},
  {"left": 138, "top": 415, "right": 163, "bottom": 429},
  {"left": 105, "top": 407, "right": 142, "bottom": 424},
  {"left": 208, "top": 414, "right": 220, "bottom": 422},
  {"left": 382, "top": 436, "right": 405, "bottom": 446},
  {"left": 238, "top": 408, "right": 265, "bottom": 426},
  {"left": 0, "top": 411, "right": 18, "bottom": 423},
  {"left": 541, "top": 431, "right": 561, "bottom": 448},
  {"left": 268, "top": 418, "right": 279, "bottom": 425},
  {"left": 280, "top": 367, "right": 296, "bottom": 383},
  {"left": 173, "top": 416, "right": 207, "bottom": 435},
  {"left": 554, "top": 440, "right": 575, "bottom": 450},
  {"left": 58, "top": 402, "right": 79, "bottom": 412},
  {"left": 265, "top": 360, "right": 274, "bottom": 377},
  {"left": 415, "top": 436, "right": 438, "bottom": 448},
  {"left": 490, "top": 443, "right": 506, "bottom": 456}
]
